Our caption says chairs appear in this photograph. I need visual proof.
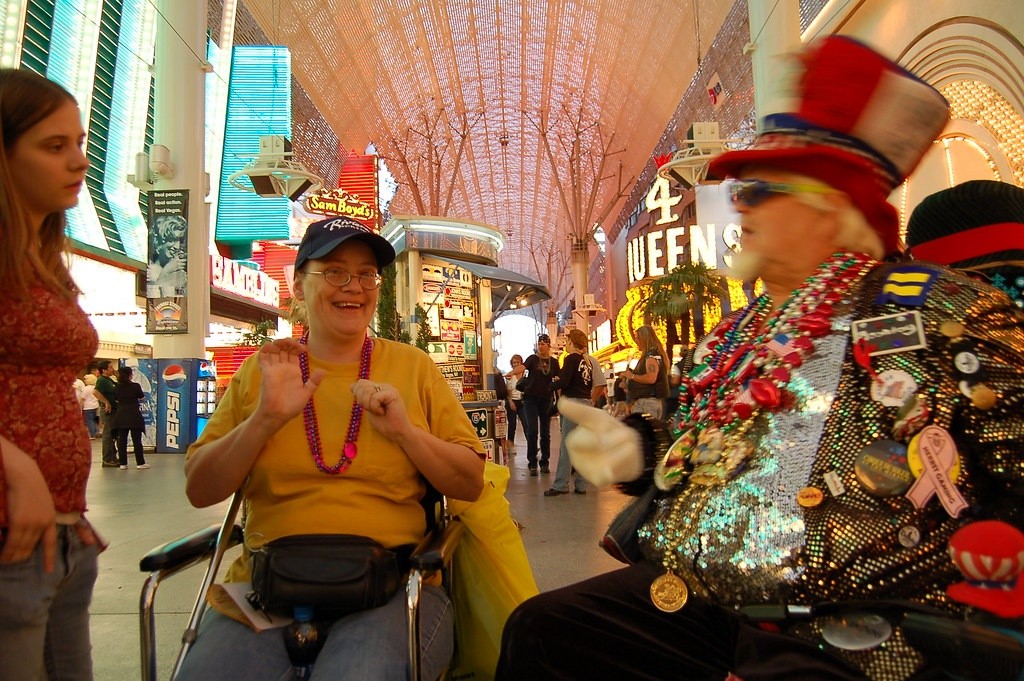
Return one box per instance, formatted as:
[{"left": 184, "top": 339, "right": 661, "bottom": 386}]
[{"left": 140, "top": 473, "right": 466, "bottom": 681}]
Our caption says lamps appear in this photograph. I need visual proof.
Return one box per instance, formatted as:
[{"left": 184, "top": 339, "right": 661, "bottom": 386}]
[
  {"left": 134, "top": 152, "right": 151, "bottom": 185},
  {"left": 147, "top": 144, "right": 177, "bottom": 180}
]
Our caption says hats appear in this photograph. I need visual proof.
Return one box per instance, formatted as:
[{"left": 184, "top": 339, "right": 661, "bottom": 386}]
[
  {"left": 566, "top": 329, "right": 587, "bottom": 347},
  {"left": 295, "top": 216, "right": 396, "bottom": 274},
  {"left": 538, "top": 334, "right": 552, "bottom": 347},
  {"left": 696, "top": 35, "right": 950, "bottom": 257},
  {"left": 905, "top": 179, "right": 1024, "bottom": 281}
]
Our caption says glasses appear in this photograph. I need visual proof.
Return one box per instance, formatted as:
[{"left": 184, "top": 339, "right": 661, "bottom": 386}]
[
  {"left": 303, "top": 267, "right": 384, "bottom": 290},
  {"left": 730, "top": 180, "right": 837, "bottom": 204}
]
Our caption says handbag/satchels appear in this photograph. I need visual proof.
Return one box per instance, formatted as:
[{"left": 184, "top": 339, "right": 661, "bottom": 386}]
[
  {"left": 246, "top": 534, "right": 396, "bottom": 617},
  {"left": 494, "top": 365, "right": 507, "bottom": 400},
  {"left": 516, "top": 377, "right": 528, "bottom": 392}
]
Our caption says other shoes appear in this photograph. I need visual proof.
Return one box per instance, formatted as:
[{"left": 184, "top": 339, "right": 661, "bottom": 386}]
[
  {"left": 540, "top": 466, "right": 551, "bottom": 473},
  {"left": 120, "top": 465, "right": 128, "bottom": 469},
  {"left": 137, "top": 464, "right": 149, "bottom": 469},
  {"left": 530, "top": 469, "right": 537, "bottom": 476},
  {"left": 90, "top": 437, "right": 95, "bottom": 440},
  {"left": 102, "top": 461, "right": 120, "bottom": 467},
  {"left": 574, "top": 489, "right": 586, "bottom": 494},
  {"left": 544, "top": 488, "right": 568, "bottom": 496}
]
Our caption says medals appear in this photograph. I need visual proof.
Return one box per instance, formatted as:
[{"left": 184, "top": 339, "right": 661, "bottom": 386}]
[{"left": 651, "top": 573, "right": 687, "bottom": 613}]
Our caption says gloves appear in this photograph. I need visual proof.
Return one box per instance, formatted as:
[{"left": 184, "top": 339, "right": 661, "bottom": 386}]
[{"left": 556, "top": 397, "right": 644, "bottom": 487}]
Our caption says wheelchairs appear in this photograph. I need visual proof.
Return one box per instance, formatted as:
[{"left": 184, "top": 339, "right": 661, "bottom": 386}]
[
  {"left": 717, "top": 598, "right": 1024, "bottom": 681},
  {"left": 139, "top": 504, "right": 466, "bottom": 681}
]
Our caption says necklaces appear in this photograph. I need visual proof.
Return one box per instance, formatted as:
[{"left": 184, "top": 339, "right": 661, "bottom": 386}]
[
  {"left": 299, "top": 330, "right": 373, "bottom": 475},
  {"left": 662, "top": 252, "right": 879, "bottom": 571},
  {"left": 539, "top": 356, "right": 550, "bottom": 375}
]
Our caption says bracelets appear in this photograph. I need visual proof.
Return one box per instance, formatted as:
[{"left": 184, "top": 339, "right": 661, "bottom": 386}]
[{"left": 630, "top": 374, "right": 634, "bottom": 380}]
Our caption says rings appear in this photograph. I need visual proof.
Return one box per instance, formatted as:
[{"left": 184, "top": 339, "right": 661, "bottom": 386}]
[
  {"left": 375, "top": 385, "right": 381, "bottom": 392},
  {"left": 180, "top": 254, "right": 184, "bottom": 258}
]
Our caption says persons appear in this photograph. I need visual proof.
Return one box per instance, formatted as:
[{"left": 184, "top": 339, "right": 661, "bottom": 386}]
[
  {"left": 73, "top": 373, "right": 104, "bottom": 440},
  {"left": 620, "top": 326, "right": 670, "bottom": 420},
  {"left": 495, "top": 36, "right": 1024, "bottom": 680},
  {"left": 146, "top": 216, "right": 186, "bottom": 297},
  {"left": 113, "top": 366, "right": 150, "bottom": 469},
  {"left": 93, "top": 361, "right": 120, "bottom": 468},
  {"left": 0, "top": 68, "right": 98, "bottom": 681},
  {"left": 543, "top": 329, "right": 607, "bottom": 496},
  {"left": 502, "top": 335, "right": 560, "bottom": 476},
  {"left": 173, "top": 216, "right": 487, "bottom": 681},
  {"left": 506, "top": 355, "right": 540, "bottom": 454},
  {"left": 606, "top": 368, "right": 633, "bottom": 419}
]
